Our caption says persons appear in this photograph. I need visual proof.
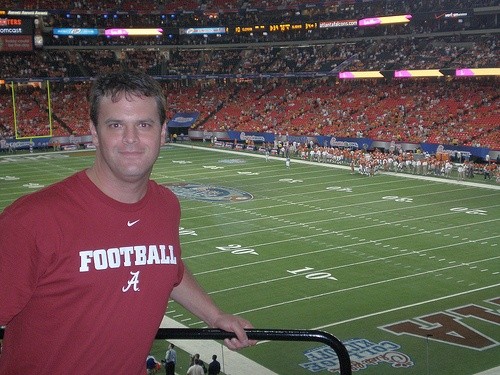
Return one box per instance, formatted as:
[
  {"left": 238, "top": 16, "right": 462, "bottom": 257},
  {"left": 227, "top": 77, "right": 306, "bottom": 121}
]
[
  {"left": 0, "top": 0, "right": 500, "bottom": 181},
  {"left": 0, "top": 65, "right": 257, "bottom": 375}
]
